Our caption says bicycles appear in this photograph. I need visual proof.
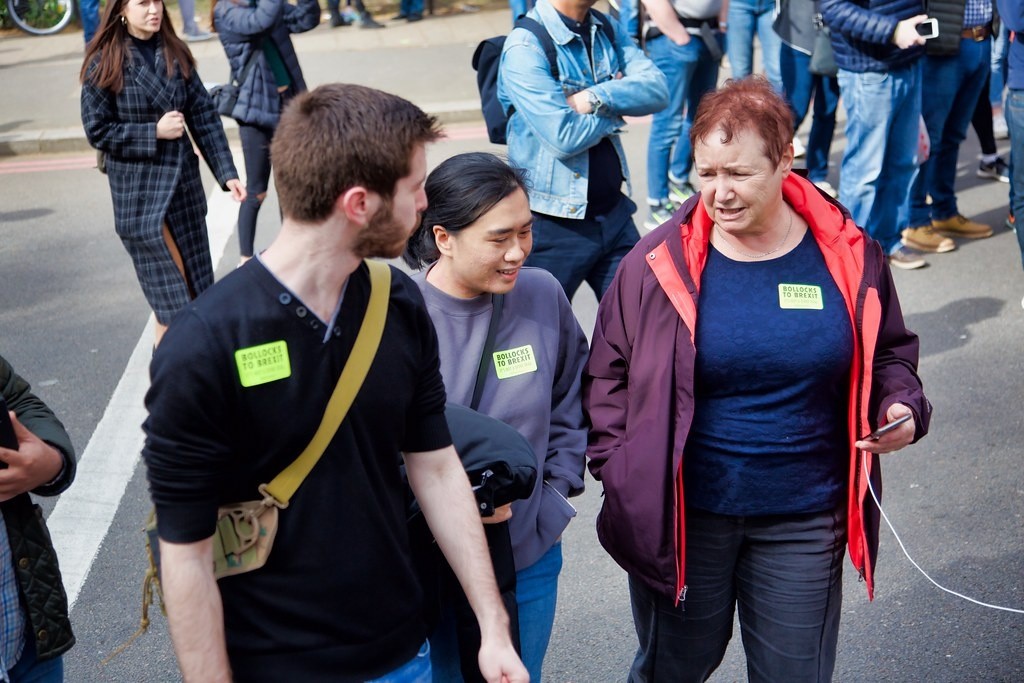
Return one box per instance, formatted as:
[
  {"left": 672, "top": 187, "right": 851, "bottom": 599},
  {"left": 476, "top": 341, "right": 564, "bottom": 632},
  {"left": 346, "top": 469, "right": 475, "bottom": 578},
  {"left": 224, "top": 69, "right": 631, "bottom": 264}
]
[{"left": 7, "top": 0, "right": 81, "bottom": 37}]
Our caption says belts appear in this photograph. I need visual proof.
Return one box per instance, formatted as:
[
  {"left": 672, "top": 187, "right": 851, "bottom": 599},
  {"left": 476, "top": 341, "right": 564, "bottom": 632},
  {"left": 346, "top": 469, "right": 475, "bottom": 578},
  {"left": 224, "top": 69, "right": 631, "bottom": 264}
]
[{"left": 959, "top": 25, "right": 993, "bottom": 42}]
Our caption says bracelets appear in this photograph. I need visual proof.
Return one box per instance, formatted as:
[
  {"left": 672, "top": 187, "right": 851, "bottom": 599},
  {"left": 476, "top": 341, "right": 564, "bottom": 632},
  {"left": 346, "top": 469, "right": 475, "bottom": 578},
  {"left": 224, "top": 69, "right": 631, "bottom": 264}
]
[{"left": 45, "top": 457, "right": 67, "bottom": 486}]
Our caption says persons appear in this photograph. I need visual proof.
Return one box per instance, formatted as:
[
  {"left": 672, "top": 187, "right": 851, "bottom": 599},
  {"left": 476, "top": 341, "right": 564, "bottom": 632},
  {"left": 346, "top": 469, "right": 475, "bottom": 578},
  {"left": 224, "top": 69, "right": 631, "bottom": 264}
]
[
  {"left": 80, "top": 0, "right": 100, "bottom": 45},
  {"left": 327, "top": 0, "right": 385, "bottom": 29},
  {"left": 139, "top": 83, "right": 530, "bottom": 683},
  {"left": 80, "top": 0, "right": 248, "bottom": 354},
  {"left": 0, "top": 355, "right": 77, "bottom": 683},
  {"left": 587, "top": 81, "right": 934, "bottom": 683},
  {"left": 392, "top": 0, "right": 426, "bottom": 22},
  {"left": 497, "top": 1, "right": 669, "bottom": 308},
  {"left": 638, "top": 0, "right": 727, "bottom": 232},
  {"left": 820, "top": 0, "right": 1024, "bottom": 269},
  {"left": 403, "top": 152, "right": 589, "bottom": 683},
  {"left": 722, "top": 0, "right": 839, "bottom": 198},
  {"left": 214, "top": 0, "right": 321, "bottom": 269}
]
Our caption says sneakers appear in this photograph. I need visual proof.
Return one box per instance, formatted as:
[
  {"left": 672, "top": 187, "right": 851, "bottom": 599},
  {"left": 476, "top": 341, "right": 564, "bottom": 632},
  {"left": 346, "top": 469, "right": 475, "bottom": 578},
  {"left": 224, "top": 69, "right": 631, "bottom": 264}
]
[
  {"left": 883, "top": 246, "right": 926, "bottom": 270},
  {"left": 650, "top": 198, "right": 676, "bottom": 225},
  {"left": 931, "top": 213, "right": 994, "bottom": 239},
  {"left": 977, "top": 155, "right": 1010, "bottom": 183},
  {"left": 899, "top": 225, "right": 955, "bottom": 252},
  {"left": 666, "top": 180, "right": 697, "bottom": 203}
]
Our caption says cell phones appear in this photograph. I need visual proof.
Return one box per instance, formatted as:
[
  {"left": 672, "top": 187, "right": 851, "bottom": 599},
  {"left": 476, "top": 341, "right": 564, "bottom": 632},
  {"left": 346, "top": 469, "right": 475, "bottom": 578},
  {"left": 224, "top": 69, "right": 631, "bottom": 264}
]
[
  {"left": 916, "top": 18, "right": 939, "bottom": 39},
  {"left": 874, "top": 413, "right": 911, "bottom": 436}
]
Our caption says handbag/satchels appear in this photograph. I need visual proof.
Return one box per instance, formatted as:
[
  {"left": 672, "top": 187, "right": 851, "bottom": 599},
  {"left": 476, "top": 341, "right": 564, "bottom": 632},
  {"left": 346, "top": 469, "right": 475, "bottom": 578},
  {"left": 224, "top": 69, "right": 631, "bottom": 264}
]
[
  {"left": 808, "top": 12, "right": 840, "bottom": 78},
  {"left": 917, "top": 114, "right": 930, "bottom": 165},
  {"left": 210, "top": 83, "right": 240, "bottom": 117}
]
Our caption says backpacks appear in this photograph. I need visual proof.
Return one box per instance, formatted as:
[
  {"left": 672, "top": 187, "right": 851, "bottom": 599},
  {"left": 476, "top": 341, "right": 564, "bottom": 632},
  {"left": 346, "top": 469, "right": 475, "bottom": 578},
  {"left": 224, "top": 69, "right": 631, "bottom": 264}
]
[{"left": 471, "top": 8, "right": 618, "bottom": 147}]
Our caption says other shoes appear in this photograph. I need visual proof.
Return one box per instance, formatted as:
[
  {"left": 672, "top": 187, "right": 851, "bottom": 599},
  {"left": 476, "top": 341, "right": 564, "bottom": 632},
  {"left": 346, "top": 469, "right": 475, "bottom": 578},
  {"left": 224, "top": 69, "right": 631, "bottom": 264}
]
[
  {"left": 1006, "top": 212, "right": 1017, "bottom": 233},
  {"left": 408, "top": 13, "right": 423, "bottom": 21},
  {"left": 358, "top": 12, "right": 385, "bottom": 28},
  {"left": 183, "top": 29, "right": 211, "bottom": 40},
  {"left": 332, "top": 14, "right": 351, "bottom": 26},
  {"left": 990, "top": 108, "right": 1008, "bottom": 138},
  {"left": 788, "top": 134, "right": 806, "bottom": 158},
  {"left": 392, "top": 14, "right": 408, "bottom": 19},
  {"left": 814, "top": 182, "right": 838, "bottom": 199}
]
